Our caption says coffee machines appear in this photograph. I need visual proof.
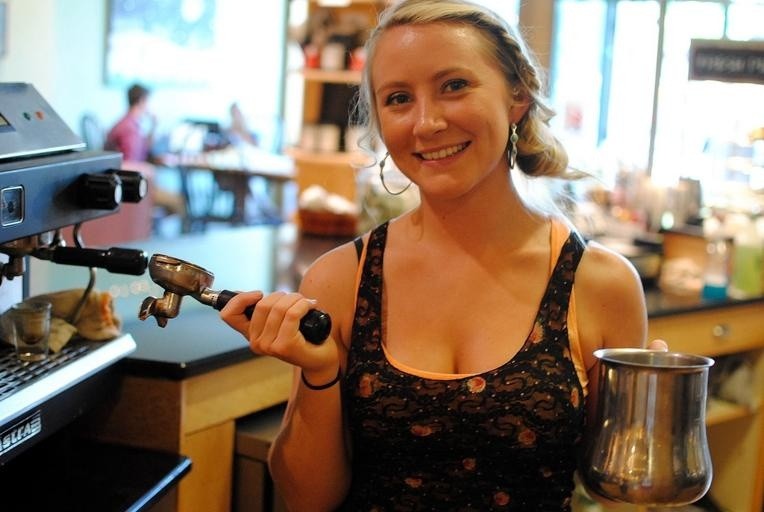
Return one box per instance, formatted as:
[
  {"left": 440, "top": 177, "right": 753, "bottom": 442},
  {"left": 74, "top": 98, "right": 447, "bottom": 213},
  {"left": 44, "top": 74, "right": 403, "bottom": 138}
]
[{"left": 1, "top": 102, "right": 195, "bottom": 511}]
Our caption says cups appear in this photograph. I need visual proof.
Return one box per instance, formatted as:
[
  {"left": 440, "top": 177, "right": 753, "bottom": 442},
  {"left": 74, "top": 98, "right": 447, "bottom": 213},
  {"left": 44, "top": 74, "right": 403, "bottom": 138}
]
[
  {"left": 9, "top": 300, "right": 53, "bottom": 362},
  {"left": 578, "top": 346, "right": 716, "bottom": 508}
]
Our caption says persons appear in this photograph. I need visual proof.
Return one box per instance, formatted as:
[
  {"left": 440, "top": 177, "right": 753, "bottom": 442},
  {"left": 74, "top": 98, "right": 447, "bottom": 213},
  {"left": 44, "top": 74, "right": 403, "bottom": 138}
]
[
  {"left": 105, "top": 83, "right": 183, "bottom": 217},
  {"left": 218, "top": 3, "right": 672, "bottom": 512},
  {"left": 212, "top": 100, "right": 260, "bottom": 225}
]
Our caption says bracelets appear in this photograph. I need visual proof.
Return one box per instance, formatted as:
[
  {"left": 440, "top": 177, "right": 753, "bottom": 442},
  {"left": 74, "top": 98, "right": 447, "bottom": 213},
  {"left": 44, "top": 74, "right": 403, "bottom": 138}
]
[{"left": 299, "top": 366, "right": 342, "bottom": 391}]
